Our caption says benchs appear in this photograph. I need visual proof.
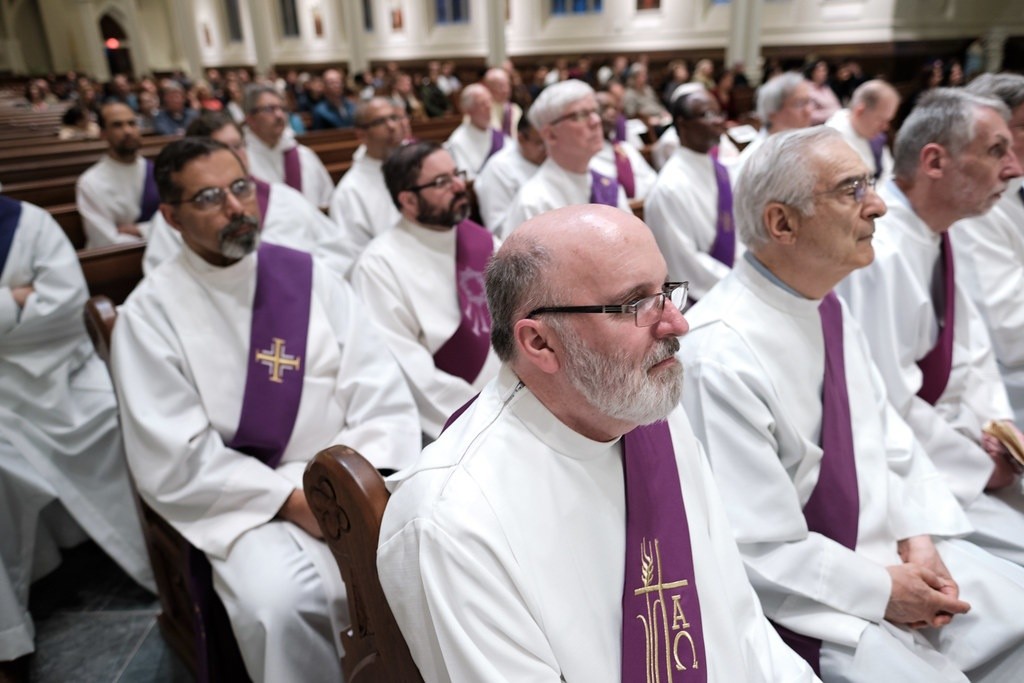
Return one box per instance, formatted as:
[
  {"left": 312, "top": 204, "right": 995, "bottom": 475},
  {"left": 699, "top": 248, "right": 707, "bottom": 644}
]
[{"left": 0, "top": 105, "right": 654, "bottom": 683}]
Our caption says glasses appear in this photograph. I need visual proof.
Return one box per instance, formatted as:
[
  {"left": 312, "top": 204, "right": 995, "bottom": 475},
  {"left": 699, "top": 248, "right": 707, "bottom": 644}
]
[
  {"left": 802, "top": 175, "right": 878, "bottom": 202},
  {"left": 166, "top": 176, "right": 251, "bottom": 208},
  {"left": 550, "top": 108, "right": 601, "bottom": 125},
  {"left": 361, "top": 114, "right": 402, "bottom": 129},
  {"left": 523, "top": 281, "right": 690, "bottom": 329},
  {"left": 412, "top": 170, "right": 467, "bottom": 193}
]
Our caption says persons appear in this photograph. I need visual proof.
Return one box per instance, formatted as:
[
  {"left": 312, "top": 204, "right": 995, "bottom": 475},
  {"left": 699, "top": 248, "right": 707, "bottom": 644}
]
[{"left": 0, "top": 42, "right": 1023, "bottom": 683}]
